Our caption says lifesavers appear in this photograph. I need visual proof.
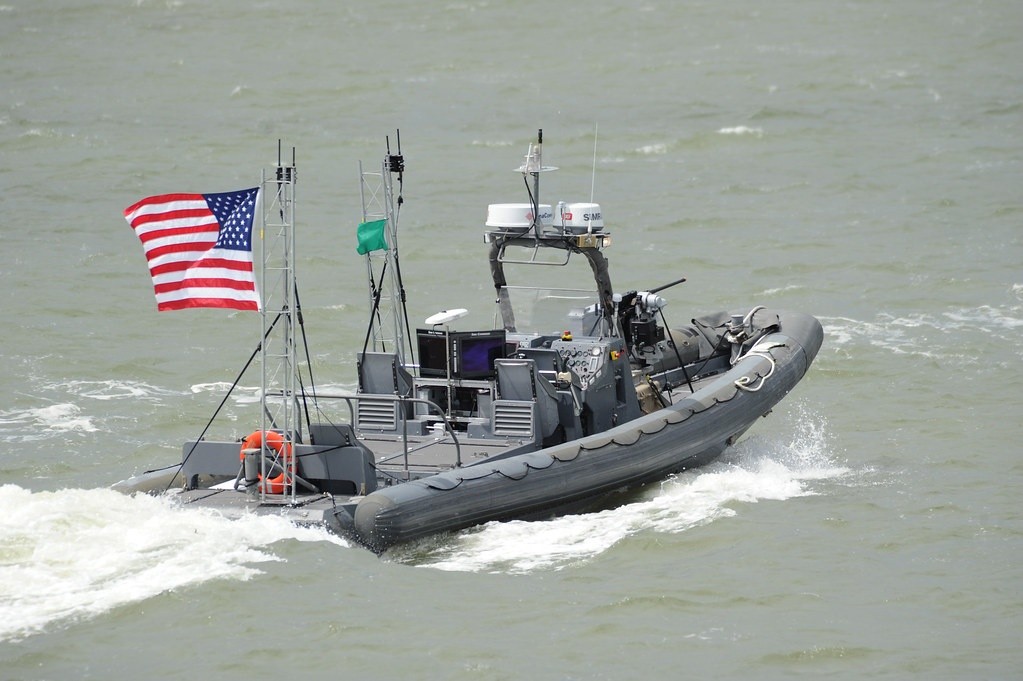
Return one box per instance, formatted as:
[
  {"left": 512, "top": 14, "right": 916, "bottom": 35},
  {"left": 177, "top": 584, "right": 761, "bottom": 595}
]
[{"left": 239, "top": 431, "right": 296, "bottom": 494}]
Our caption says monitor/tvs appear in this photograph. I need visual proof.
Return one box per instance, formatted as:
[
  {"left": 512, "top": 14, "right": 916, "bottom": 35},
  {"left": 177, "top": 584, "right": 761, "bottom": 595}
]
[{"left": 416, "top": 329, "right": 506, "bottom": 379}]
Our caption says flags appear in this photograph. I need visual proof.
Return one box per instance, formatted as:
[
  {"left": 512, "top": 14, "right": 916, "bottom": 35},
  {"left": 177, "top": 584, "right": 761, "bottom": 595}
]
[
  {"left": 356, "top": 217, "right": 391, "bottom": 256},
  {"left": 121, "top": 187, "right": 263, "bottom": 313}
]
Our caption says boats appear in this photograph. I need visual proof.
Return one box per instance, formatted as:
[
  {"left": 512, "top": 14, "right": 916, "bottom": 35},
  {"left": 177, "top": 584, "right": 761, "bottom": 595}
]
[{"left": 107, "top": 125, "right": 824, "bottom": 550}]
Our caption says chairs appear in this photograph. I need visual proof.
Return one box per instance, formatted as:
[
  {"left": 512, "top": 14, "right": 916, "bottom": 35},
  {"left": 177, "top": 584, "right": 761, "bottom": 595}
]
[
  {"left": 514, "top": 345, "right": 587, "bottom": 441},
  {"left": 253, "top": 428, "right": 304, "bottom": 444},
  {"left": 492, "top": 359, "right": 561, "bottom": 437},
  {"left": 355, "top": 351, "right": 413, "bottom": 417},
  {"left": 307, "top": 419, "right": 375, "bottom": 493}
]
